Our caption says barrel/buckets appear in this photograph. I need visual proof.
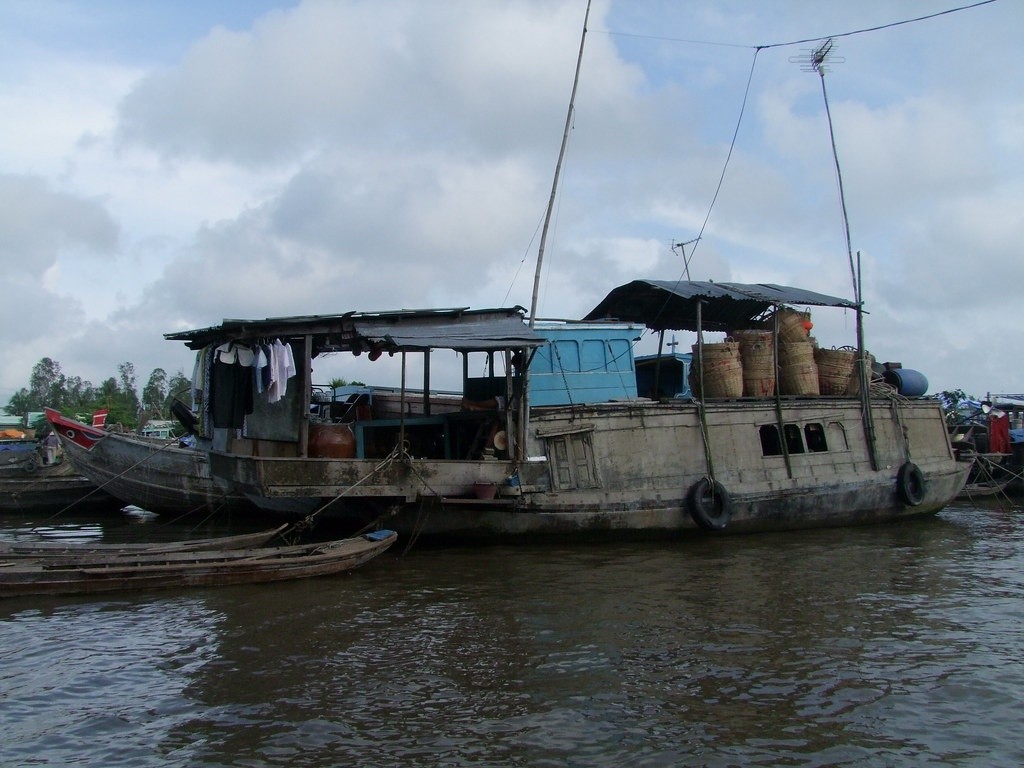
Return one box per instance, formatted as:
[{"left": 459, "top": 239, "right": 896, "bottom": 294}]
[{"left": 881, "top": 369, "right": 929, "bottom": 395}]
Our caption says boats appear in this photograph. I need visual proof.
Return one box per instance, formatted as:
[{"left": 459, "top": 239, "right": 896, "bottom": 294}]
[
  {"left": 0, "top": 530, "right": 398, "bottom": 596},
  {"left": 0, "top": 523, "right": 288, "bottom": 555},
  {"left": 955, "top": 477, "right": 1010, "bottom": 498},
  {"left": 945, "top": 422, "right": 1016, "bottom": 483},
  {"left": 43, "top": 305, "right": 975, "bottom": 546},
  {"left": 10, "top": 422, "right": 114, "bottom": 509}
]
